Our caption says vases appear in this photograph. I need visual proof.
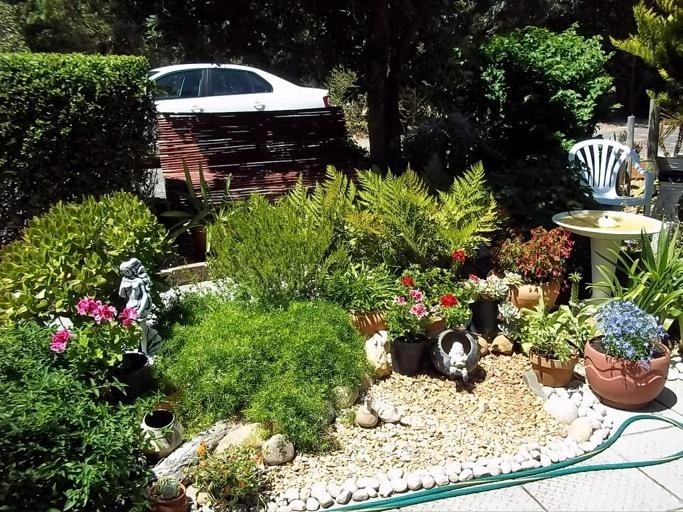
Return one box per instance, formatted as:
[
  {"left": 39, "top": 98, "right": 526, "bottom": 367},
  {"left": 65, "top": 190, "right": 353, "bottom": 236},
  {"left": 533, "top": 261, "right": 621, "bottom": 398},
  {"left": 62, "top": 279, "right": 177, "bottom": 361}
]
[
  {"left": 431, "top": 330, "right": 479, "bottom": 382},
  {"left": 139, "top": 408, "right": 183, "bottom": 458},
  {"left": 423, "top": 316, "right": 444, "bottom": 341},
  {"left": 389, "top": 335, "right": 430, "bottom": 377},
  {"left": 583, "top": 336, "right": 670, "bottom": 410},
  {"left": 176, "top": 230, "right": 205, "bottom": 260},
  {"left": 464, "top": 300, "right": 500, "bottom": 334},
  {"left": 487, "top": 267, "right": 560, "bottom": 314},
  {"left": 101, "top": 353, "right": 150, "bottom": 401}
]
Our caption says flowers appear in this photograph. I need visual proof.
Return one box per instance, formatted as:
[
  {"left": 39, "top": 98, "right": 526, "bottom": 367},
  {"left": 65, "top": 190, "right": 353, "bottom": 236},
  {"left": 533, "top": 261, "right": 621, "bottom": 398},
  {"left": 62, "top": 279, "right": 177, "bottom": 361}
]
[
  {"left": 50, "top": 294, "right": 145, "bottom": 368},
  {"left": 156, "top": 158, "right": 234, "bottom": 251},
  {"left": 453, "top": 270, "right": 523, "bottom": 304},
  {"left": 488, "top": 226, "right": 569, "bottom": 290},
  {"left": 394, "top": 249, "right": 472, "bottom": 330},
  {"left": 382, "top": 290, "right": 431, "bottom": 342},
  {"left": 587, "top": 301, "right": 669, "bottom": 374}
]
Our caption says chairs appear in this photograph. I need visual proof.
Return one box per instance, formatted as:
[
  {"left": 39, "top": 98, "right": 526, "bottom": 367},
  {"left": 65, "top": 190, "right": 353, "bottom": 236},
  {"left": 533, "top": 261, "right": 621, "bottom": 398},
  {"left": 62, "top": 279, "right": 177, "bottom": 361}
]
[{"left": 568, "top": 139, "right": 654, "bottom": 216}]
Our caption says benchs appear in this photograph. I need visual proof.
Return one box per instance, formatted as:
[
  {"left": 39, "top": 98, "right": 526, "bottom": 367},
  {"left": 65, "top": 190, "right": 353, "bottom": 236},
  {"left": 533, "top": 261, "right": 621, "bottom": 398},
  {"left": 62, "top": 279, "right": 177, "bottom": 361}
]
[{"left": 149, "top": 107, "right": 367, "bottom": 211}]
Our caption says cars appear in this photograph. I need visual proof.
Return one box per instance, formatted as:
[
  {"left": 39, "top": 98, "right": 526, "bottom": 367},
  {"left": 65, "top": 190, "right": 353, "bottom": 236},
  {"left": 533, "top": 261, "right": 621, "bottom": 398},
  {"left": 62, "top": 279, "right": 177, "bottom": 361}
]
[{"left": 147, "top": 64, "right": 329, "bottom": 164}]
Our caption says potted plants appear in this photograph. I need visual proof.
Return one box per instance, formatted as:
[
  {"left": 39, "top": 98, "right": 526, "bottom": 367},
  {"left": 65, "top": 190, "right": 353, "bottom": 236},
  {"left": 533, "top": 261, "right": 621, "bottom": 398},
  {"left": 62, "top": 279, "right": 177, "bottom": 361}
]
[
  {"left": 149, "top": 476, "right": 188, "bottom": 512},
  {"left": 325, "top": 260, "right": 394, "bottom": 335},
  {"left": 518, "top": 305, "right": 579, "bottom": 386}
]
